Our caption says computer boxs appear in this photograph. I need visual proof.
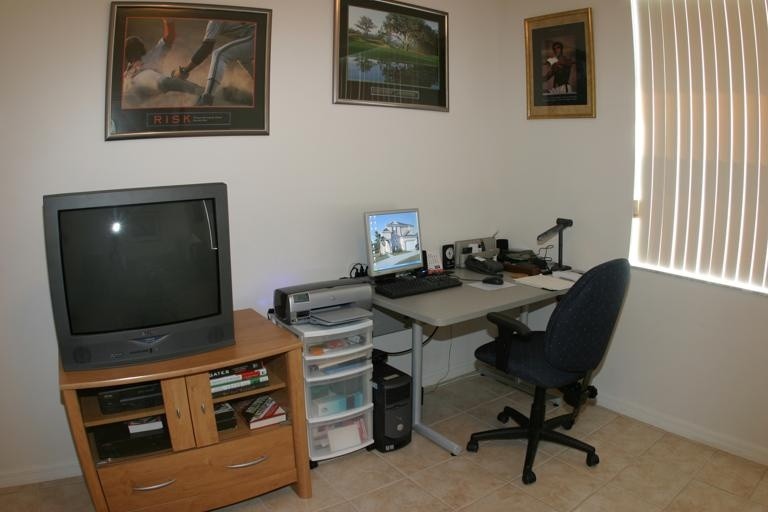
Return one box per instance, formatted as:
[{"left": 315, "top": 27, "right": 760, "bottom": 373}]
[{"left": 366, "top": 356, "right": 413, "bottom": 454}]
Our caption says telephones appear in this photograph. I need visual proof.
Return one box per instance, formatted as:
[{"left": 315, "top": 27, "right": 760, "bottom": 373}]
[{"left": 464, "top": 254, "right": 504, "bottom": 278}]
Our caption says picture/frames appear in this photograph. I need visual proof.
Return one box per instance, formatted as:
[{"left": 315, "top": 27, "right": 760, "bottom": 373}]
[
  {"left": 103, "top": 1, "right": 274, "bottom": 142},
  {"left": 522, "top": 6, "right": 597, "bottom": 122},
  {"left": 331, "top": 0, "right": 451, "bottom": 114}
]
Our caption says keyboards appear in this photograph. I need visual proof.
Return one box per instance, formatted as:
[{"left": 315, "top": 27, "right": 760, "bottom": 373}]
[{"left": 376, "top": 274, "right": 463, "bottom": 299}]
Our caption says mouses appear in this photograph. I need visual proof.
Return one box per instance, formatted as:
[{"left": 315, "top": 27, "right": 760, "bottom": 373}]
[{"left": 482, "top": 276, "right": 504, "bottom": 286}]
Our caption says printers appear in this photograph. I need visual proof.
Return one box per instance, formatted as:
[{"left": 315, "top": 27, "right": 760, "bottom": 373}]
[{"left": 273, "top": 277, "right": 376, "bottom": 326}]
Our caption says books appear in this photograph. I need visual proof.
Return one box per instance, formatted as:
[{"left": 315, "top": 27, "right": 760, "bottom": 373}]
[
  {"left": 129, "top": 414, "right": 164, "bottom": 434},
  {"left": 209, "top": 362, "right": 287, "bottom": 432}
]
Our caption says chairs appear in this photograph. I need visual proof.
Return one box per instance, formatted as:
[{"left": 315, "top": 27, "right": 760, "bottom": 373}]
[{"left": 465, "top": 257, "right": 634, "bottom": 486}]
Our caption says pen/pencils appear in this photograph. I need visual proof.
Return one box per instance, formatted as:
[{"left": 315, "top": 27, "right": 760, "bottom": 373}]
[{"left": 559, "top": 276, "right": 576, "bottom": 283}]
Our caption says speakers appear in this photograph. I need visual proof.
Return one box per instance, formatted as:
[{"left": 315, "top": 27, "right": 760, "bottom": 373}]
[
  {"left": 442, "top": 244, "right": 455, "bottom": 270},
  {"left": 496, "top": 238, "right": 509, "bottom": 262}
]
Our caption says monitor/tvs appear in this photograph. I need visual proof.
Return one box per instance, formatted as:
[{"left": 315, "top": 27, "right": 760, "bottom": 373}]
[
  {"left": 42, "top": 182, "right": 236, "bottom": 372},
  {"left": 363, "top": 208, "right": 424, "bottom": 285}
]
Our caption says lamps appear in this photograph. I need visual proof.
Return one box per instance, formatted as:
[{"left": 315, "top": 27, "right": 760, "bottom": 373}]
[{"left": 534, "top": 215, "right": 576, "bottom": 273}]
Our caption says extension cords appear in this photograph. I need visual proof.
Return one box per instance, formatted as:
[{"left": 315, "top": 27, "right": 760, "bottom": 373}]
[{"left": 340, "top": 276, "right": 370, "bottom": 283}]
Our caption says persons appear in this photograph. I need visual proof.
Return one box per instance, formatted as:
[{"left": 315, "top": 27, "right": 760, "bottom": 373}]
[
  {"left": 537, "top": 42, "right": 570, "bottom": 98},
  {"left": 123, "top": 17, "right": 204, "bottom": 104},
  {"left": 179, "top": 18, "right": 253, "bottom": 111}
]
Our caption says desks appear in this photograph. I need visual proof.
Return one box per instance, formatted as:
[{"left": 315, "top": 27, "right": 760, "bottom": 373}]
[{"left": 372, "top": 266, "right": 594, "bottom": 459}]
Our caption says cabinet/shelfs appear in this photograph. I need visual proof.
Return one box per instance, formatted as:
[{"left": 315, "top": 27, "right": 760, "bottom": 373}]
[
  {"left": 57, "top": 305, "right": 313, "bottom": 512},
  {"left": 266, "top": 307, "right": 378, "bottom": 471}
]
